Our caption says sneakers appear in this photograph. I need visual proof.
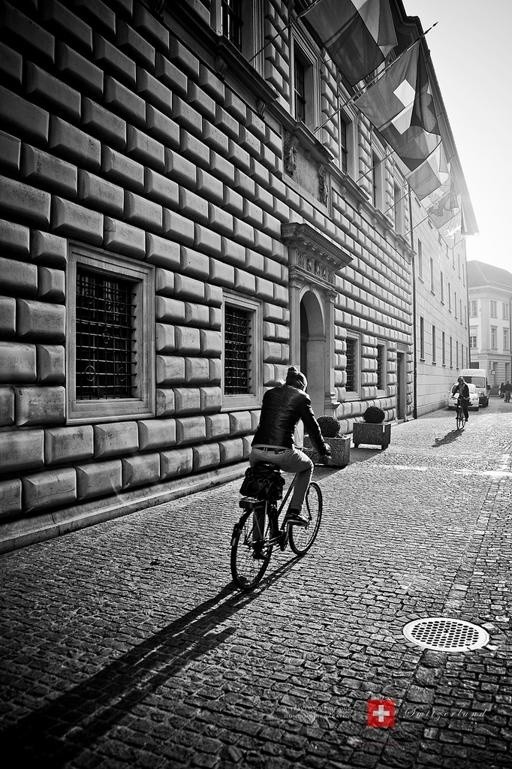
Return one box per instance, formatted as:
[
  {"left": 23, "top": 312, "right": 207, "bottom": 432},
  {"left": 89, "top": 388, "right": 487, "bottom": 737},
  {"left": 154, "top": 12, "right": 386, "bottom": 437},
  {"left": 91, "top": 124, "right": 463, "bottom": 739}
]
[
  {"left": 287, "top": 514, "right": 309, "bottom": 526},
  {"left": 252, "top": 550, "right": 267, "bottom": 560}
]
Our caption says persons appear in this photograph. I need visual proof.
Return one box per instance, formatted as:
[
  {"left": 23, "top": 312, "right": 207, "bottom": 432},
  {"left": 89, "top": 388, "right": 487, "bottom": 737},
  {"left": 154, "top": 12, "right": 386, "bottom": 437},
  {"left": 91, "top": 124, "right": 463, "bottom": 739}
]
[
  {"left": 499, "top": 382, "right": 504, "bottom": 397},
  {"left": 246, "top": 363, "right": 332, "bottom": 560},
  {"left": 452, "top": 376, "right": 471, "bottom": 423},
  {"left": 503, "top": 380, "right": 511, "bottom": 402}
]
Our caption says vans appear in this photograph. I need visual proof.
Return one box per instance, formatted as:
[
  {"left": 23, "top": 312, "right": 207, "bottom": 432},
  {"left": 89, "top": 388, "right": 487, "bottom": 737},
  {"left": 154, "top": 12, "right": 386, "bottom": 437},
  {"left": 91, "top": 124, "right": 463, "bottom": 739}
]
[{"left": 457, "top": 368, "right": 492, "bottom": 407}]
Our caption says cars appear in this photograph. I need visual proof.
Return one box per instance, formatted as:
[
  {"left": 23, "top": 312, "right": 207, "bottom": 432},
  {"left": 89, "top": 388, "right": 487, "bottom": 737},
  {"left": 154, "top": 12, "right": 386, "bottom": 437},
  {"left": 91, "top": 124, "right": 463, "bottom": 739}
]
[{"left": 446, "top": 383, "right": 481, "bottom": 412}]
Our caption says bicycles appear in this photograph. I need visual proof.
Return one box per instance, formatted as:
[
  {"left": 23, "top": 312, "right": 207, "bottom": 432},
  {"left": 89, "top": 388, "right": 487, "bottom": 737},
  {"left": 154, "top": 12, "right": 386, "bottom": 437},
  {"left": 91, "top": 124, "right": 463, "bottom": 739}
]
[
  {"left": 223, "top": 444, "right": 336, "bottom": 594},
  {"left": 451, "top": 397, "right": 469, "bottom": 432},
  {"left": 504, "top": 391, "right": 510, "bottom": 403}
]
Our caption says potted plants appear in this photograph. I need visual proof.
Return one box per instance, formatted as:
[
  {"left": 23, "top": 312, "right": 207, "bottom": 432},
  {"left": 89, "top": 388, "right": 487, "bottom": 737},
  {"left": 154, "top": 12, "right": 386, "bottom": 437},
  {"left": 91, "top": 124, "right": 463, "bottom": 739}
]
[
  {"left": 351, "top": 404, "right": 392, "bottom": 450},
  {"left": 302, "top": 414, "right": 352, "bottom": 468}
]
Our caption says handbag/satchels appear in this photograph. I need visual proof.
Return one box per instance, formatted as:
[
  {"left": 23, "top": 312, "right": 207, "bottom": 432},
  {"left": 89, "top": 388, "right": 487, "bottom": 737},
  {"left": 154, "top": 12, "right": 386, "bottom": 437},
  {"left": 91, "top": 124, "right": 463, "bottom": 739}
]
[{"left": 239, "top": 466, "right": 286, "bottom": 500}]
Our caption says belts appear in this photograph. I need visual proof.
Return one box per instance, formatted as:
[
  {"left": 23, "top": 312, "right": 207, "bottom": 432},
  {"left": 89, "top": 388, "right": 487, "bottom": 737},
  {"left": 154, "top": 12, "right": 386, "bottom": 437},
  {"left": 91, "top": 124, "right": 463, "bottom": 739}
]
[{"left": 255, "top": 446, "right": 287, "bottom": 452}]
